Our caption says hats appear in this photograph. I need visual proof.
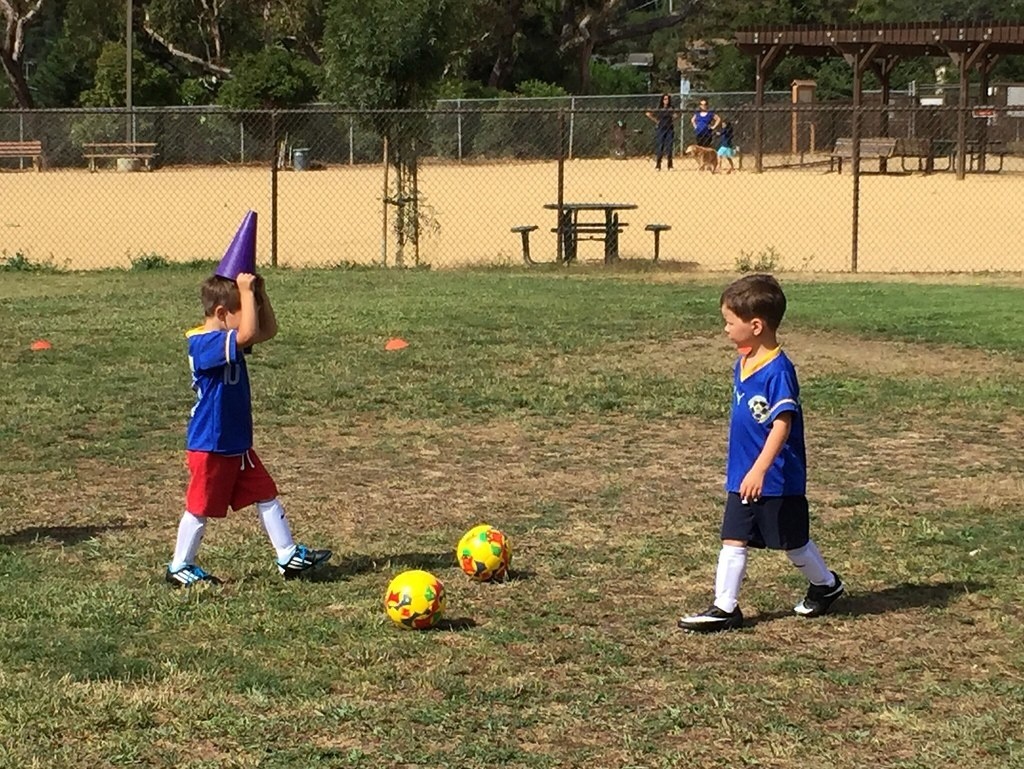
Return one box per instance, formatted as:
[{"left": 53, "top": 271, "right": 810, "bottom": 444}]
[{"left": 214, "top": 210, "right": 258, "bottom": 281}]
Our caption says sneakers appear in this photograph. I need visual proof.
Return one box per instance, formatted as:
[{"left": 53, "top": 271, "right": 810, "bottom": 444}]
[
  {"left": 678, "top": 605, "right": 744, "bottom": 633},
  {"left": 277, "top": 546, "right": 332, "bottom": 580},
  {"left": 165, "top": 566, "right": 222, "bottom": 587},
  {"left": 792, "top": 570, "right": 845, "bottom": 617}
]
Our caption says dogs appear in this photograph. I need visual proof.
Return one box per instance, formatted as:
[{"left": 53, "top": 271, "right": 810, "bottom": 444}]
[{"left": 685, "top": 144, "right": 719, "bottom": 175}]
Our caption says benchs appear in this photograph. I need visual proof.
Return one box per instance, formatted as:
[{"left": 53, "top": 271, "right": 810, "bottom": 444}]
[
  {"left": 81, "top": 143, "right": 160, "bottom": 174},
  {"left": 0, "top": 141, "right": 42, "bottom": 173},
  {"left": 825, "top": 137, "right": 1014, "bottom": 174},
  {"left": 511, "top": 226, "right": 539, "bottom": 266},
  {"left": 645, "top": 225, "right": 671, "bottom": 259}
]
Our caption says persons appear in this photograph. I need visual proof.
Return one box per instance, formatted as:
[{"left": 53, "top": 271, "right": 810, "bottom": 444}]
[
  {"left": 677, "top": 275, "right": 843, "bottom": 631},
  {"left": 646, "top": 94, "right": 683, "bottom": 171},
  {"left": 691, "top": 99, "right": 720, "bottom": 146},
  {"left": 715, "top": 120, "right": 734, "bottom": 171},
  {"left": 166, "top": 273, "right": 333, "bottom": 588}
]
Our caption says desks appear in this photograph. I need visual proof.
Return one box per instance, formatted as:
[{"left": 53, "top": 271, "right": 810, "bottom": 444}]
[{"left": 543, "top": 203, "right": 637, "bottom": 264}]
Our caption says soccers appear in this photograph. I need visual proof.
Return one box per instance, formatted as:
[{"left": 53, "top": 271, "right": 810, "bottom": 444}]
[
  {"left": 454, "top": 524, "right": 514, "bottom": 583},
  {"left": 381, "top": 571, "right": 449, "bottom": 626}
]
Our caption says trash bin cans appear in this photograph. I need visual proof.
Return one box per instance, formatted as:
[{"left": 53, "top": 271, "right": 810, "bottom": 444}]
[{"left": 293, "top": 147, "right": 312, "bottom": 172}]
